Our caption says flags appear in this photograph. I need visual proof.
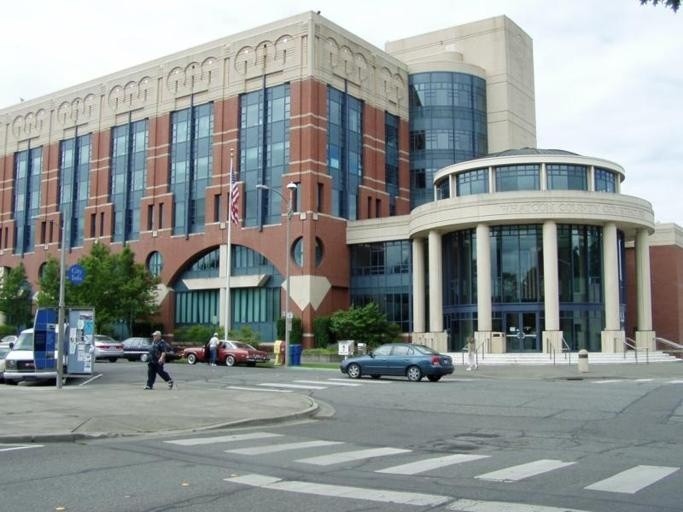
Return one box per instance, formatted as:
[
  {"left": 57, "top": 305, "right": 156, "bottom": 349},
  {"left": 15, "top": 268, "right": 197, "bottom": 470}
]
[{"left": 230, "top": 167, "right": 239, "bottom": 225}]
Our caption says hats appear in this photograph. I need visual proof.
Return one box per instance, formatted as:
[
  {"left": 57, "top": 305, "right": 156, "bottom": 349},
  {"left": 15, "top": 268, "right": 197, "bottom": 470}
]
[{"left": 152, "top": 331, "right": 161, "bottom": 336}]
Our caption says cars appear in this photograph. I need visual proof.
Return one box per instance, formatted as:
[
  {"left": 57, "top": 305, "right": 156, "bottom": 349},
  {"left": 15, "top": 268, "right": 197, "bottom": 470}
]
[
  {"left": 179, "top": 339, "right": 272, "bottom": 368},
  {"left": 338, "top": 342, "right": 453, "bottom": 383},
  {"left": 116, "top": 335, "right": 184, "bottom": 362},
  {"left": 0, "top": 331, "right": 124, "bottom": 375}
]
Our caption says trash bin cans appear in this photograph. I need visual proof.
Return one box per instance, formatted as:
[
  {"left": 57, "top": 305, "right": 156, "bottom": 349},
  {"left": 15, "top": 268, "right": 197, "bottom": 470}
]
[
  {"left": 578, "top": 349, "right": 589, "bottom": 373},
  {"left": 492, "top": 332, "right": 506, "bottom": 354},
  {"left": 290, "top": 345, "right": 302, "bottom": 365}
]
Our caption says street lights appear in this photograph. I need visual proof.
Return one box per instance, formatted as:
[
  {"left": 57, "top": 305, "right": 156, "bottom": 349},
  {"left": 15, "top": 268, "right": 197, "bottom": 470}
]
[{"left": 255, "top": 178, "right": 298, "bottom": 370}]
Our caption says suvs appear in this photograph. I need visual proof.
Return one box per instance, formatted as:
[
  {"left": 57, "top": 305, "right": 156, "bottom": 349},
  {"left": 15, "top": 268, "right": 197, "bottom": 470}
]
[{"left": 2, "top": 323, "right": 75, "bottom": 385}]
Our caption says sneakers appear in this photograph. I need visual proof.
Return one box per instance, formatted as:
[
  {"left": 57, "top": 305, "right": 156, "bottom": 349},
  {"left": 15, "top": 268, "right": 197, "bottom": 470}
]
[
  {"left": 144, "top": 386, "right": 153, "bottom": 389},
  {"left": 168, "top": 380, "right": 173, "bottom": 390}
]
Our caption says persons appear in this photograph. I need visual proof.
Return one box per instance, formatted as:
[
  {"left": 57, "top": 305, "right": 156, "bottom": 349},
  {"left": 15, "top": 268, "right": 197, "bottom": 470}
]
[
  {"left": 143, "top": 330, "right": 173, "bottom": 390},
  {"left": 206, "top": 333, "right": 220, "bottom": 366}
]
